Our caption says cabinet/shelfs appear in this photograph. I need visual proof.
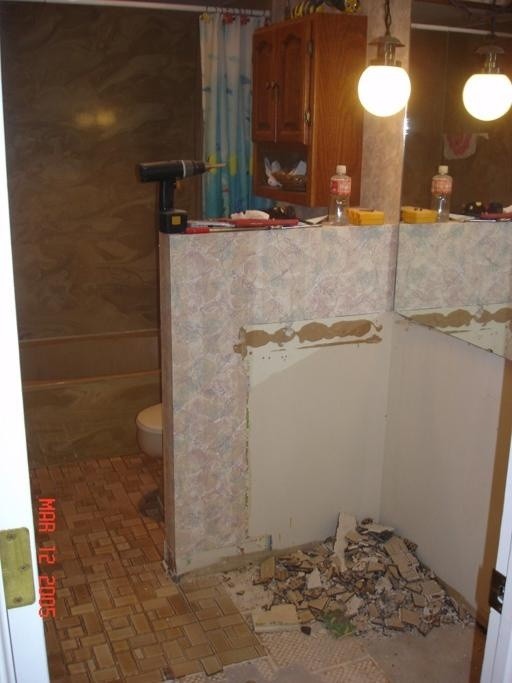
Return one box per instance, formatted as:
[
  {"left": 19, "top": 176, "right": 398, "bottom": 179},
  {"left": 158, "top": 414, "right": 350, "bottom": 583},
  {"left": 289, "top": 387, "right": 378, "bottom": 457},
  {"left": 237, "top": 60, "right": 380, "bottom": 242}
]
[{"left": 246, "top": 9, "right": 367, "bottom": 211}]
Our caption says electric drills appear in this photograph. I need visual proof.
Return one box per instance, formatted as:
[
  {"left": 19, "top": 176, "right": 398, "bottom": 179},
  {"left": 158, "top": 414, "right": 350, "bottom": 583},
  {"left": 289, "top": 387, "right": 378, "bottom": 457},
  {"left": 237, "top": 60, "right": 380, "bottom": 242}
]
[{"left": 136, "top": 159, "right": 226, "bottom": 232}]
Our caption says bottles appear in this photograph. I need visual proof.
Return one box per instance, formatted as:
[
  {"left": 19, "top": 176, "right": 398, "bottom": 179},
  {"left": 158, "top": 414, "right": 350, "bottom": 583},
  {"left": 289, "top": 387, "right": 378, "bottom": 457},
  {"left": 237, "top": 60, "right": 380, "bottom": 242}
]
[
  {"left": 431, "top": 165, "right": 454, "bottom": 223},
  {"left": 327, "top": 162, "right": 352, "bottom": 225}
]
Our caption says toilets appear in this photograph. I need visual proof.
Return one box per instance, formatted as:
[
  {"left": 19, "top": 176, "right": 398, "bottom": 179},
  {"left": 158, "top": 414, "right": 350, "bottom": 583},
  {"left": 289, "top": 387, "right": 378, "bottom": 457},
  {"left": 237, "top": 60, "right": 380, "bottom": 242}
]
[{"left": 135, "top": 403, "right": 163, "bottom": 458}]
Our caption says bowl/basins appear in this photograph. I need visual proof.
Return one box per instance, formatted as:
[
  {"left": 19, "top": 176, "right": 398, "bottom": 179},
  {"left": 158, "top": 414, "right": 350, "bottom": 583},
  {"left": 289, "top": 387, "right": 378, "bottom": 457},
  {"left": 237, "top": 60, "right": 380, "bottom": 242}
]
[{"left": 271, "top": 169, "right": 306, "bottom": 190}]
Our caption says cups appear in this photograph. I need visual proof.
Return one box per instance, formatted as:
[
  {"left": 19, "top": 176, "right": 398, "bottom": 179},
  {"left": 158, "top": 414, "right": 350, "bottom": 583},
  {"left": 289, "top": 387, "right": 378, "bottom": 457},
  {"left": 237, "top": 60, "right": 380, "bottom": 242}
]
[{"left": 265, "top": 206, "right": 286, "bottom": 229}]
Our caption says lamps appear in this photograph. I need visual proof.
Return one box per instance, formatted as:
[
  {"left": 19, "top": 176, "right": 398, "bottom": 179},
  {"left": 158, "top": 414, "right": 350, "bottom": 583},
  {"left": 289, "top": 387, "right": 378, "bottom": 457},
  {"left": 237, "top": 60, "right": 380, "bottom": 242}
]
[
  {"left": 352, "top": 0, "right": 411, "bottom": 120},
  {"left": 457, "top": 1, "right": 512, "bottom": 124}
]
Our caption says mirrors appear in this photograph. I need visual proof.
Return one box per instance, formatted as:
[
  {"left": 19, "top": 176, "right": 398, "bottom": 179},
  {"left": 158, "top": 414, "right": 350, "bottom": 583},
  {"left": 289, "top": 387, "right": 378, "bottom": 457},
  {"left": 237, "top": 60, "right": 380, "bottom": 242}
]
[{"left": 394, "top": 0, "right": 512, "bottom": 365}]
